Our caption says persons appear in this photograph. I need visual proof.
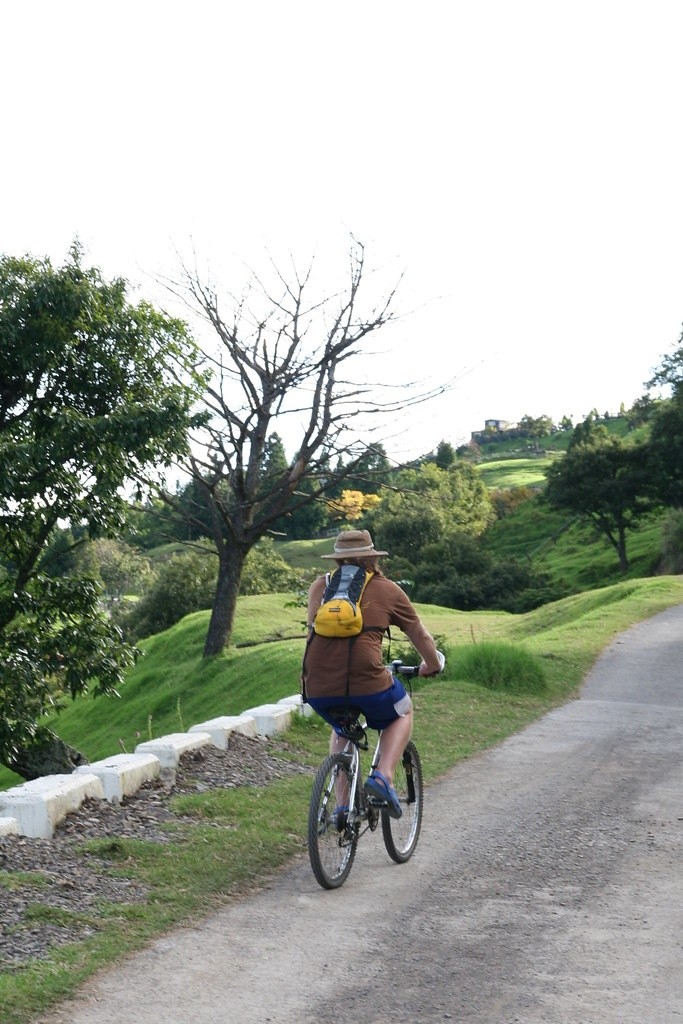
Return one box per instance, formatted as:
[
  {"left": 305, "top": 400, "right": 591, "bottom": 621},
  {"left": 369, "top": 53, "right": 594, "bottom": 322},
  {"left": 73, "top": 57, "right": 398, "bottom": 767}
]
[{"left": 301, "top": 529, "right": 445, "bottom": 830}]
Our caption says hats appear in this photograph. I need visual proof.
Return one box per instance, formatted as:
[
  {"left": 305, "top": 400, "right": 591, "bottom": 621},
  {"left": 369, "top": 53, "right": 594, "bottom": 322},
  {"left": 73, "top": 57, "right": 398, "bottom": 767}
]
[{"left": 320, "top": 530, "right": 389, "bottom": 559}]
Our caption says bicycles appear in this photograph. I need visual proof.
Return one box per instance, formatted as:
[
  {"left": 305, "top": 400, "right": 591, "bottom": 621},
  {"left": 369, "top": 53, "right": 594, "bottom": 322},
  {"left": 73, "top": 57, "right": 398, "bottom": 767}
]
[{"left": 307, "top": 660, "right": 442, "bottom": 888}]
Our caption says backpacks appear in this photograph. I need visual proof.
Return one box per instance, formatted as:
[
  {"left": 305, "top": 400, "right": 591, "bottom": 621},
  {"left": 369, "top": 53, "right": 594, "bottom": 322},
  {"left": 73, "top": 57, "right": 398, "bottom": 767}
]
[{"left": 310, "top": 563, "right": 379, "bottom": 637}]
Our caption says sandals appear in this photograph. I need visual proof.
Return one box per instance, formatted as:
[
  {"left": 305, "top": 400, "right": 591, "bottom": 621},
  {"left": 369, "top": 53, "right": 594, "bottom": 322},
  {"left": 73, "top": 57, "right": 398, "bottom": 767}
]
[
  {"left": 333, "top": 806, "right": 348, "bottom": 814},
  {"left": 363, "top": 770, "right": 402, "bottom": 819}
]
